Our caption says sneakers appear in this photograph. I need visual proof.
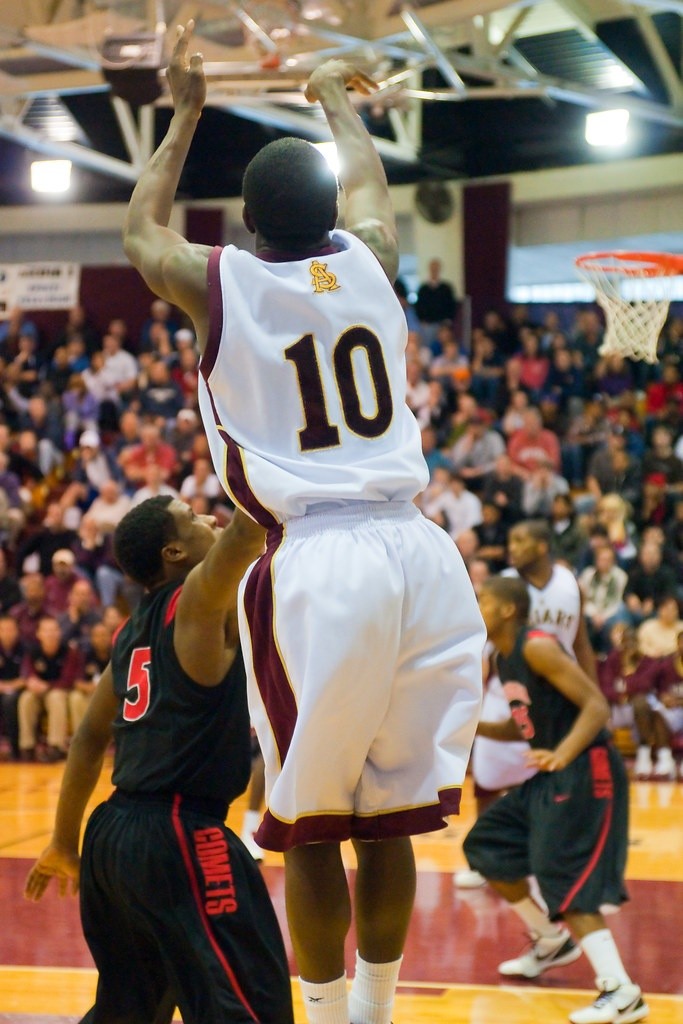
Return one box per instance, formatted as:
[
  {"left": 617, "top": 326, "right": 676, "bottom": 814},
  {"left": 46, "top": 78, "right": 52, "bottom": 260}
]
[
  {"left": 498, "top": 928, "right": 582, "bottom": 978},
  {"left": 568, "top": 979, "right": 649, "bottom": 1024}
]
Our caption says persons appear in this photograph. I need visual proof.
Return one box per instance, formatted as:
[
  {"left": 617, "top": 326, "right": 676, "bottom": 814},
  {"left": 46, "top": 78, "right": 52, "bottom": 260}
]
[
  {"left": 0, "top": 258, "right": 683, "bottom": 1024},
  {"left": 121, "top": 18, "right": 488, "bottom": 1024}
]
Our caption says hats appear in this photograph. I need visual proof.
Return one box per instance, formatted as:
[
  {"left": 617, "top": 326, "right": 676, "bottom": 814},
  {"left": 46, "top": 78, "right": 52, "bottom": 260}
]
[
  {"left": 453, "top": 367, "right": 471, "bottom": 380},
  {"left": 79, "top": 430, "right": 101, "bottom": 446},
  {"left": 52, "top": 549, "right": 77, "bottom": 565},
  {"left": 21, "top": 323, "right": 37, "bottom": 337},
  {"left": 176, "top": 328, "right": 195, "bottom": 341},
  {"left": 613, "top": 424, "right": 627, "bottom": 440}
]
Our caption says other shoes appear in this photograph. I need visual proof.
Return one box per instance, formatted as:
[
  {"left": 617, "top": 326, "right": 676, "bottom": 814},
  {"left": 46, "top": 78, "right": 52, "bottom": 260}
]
[
  {"left": 244, "top": 832, "right": 265, "bottom": 863},
  {"left": 635, "top": 747, "right": 653, "bottom": 778},
  {"left": 454, "top": 868, "right": 485, "bottom": 887},
  {"left": 18, "top": 746, "right": 63, "bottom": 762},
  {"left": 654, "top": 748, "right": 676, "bottom": 778}
]
